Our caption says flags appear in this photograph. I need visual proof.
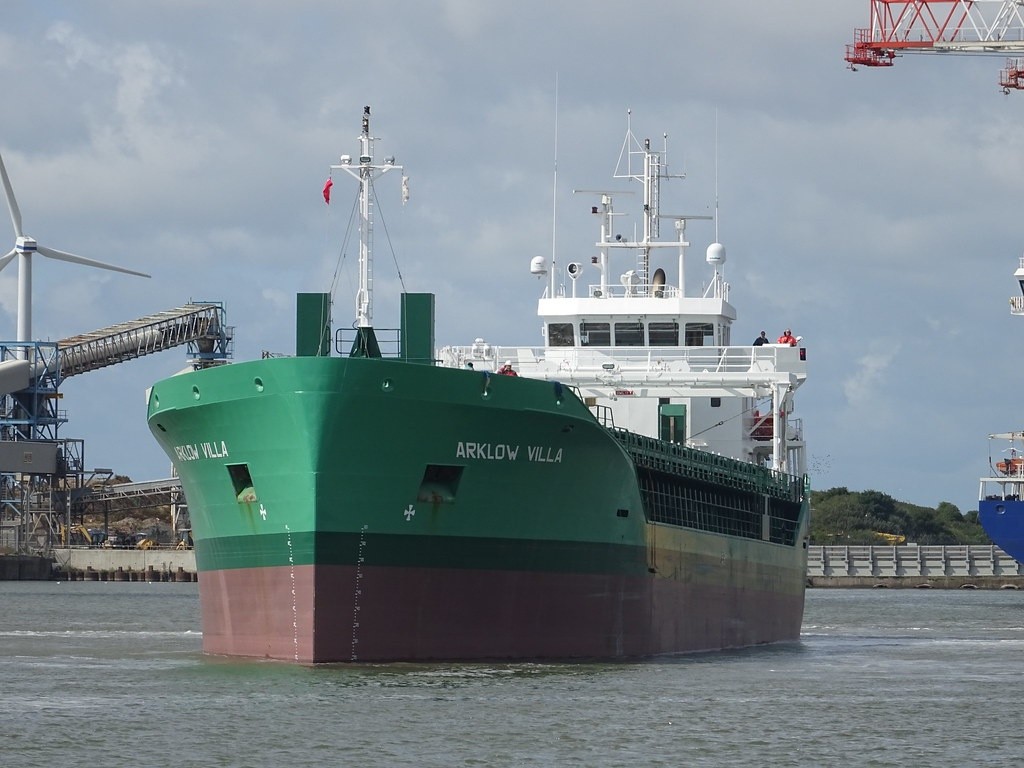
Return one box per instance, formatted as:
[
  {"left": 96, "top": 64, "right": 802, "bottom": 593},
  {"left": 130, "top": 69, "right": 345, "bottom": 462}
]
[{"left": 322, "top": 179, "right": 333, "bottom": 205}]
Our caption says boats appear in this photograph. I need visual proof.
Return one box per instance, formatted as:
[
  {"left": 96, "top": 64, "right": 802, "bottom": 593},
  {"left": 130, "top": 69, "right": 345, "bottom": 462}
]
[
  {"left": 146, "top": 108, "right": 812, "bottom": 661},
  {"left": 979, "top": 255, "right": 1024, "bottom": 568}
]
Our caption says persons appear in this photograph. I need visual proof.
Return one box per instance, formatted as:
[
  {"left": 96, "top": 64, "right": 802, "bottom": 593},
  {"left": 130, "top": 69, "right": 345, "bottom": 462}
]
[
  {"left": 497, "top": 360, "right": 518, "bottom": 377},
  {"left": 777, "top": 330, "right": 796, "bottom": 347},
  {"left": 752, "top": 331, "right": 769, "bottom": 346}
]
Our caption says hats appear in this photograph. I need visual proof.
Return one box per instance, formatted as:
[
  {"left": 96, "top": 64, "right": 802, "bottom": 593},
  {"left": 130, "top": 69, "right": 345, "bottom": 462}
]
[{"left": 505, "top": 360, "right": 511, "bottom": 366}]
